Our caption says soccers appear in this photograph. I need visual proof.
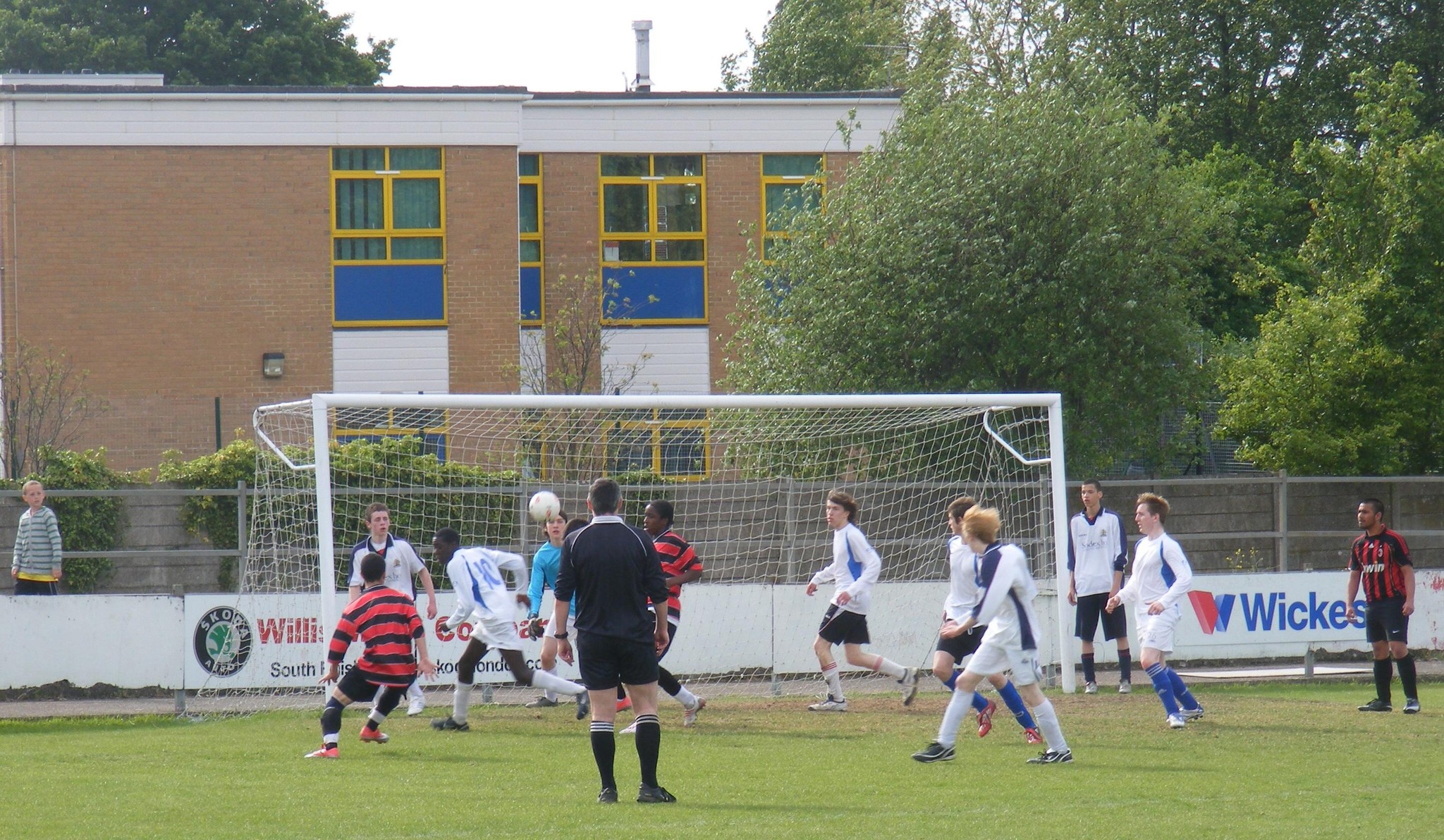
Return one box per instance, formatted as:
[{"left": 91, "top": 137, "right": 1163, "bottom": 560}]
[{"left": 530, "top": 491, "right": 562, "bottom": 523}]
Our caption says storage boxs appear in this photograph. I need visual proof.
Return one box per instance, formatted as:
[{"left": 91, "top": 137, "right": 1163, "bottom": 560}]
[{"left": 642, "top": 204, "right": 668, "bottom": 261}]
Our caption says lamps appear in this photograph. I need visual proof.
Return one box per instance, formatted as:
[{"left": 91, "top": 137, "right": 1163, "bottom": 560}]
[{"left": 262, "top": 352, "right": 285, "bottom": 379}]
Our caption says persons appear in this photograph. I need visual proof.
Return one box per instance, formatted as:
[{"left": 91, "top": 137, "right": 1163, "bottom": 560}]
[
  {"left": 553, "top": 478, "right": 677, "bottom": 804},
  {"left": 526, "top": 498, "right": 707, "bottom": 734},
  {"left": 1066, "top": 478, "right": 1132, "bottom": 696},
  {"left": 911, "top": 505, "right": 1073, "bottom": 765},
  {"left": 805, "top": 491, "right": 920, "bottom": 710},
  {"left": 1344, "top": 498, "right": 1421, "bottom": 713},
  {"left": 347, "top": 502, "right": 437, "bottom": 715},
  {"left": 10, "top": 481, "right": 62, "bottom": 595},
  {"left": 430, "top": 527, "right": 590, "bottom": 732},
  {"left": 1105, "top": 492, "right": 1204, "bottom": 729},
  {"left": 932, "top": 497, "right": 1043, "bottom": 746},
  {"left": 305, "top": 553, "right": 441, "bottom": 759}
]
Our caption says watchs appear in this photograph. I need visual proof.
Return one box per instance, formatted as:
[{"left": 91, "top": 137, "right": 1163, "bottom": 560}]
[{"left": 554, "top": 631, "right": 568, "bottom": 639}]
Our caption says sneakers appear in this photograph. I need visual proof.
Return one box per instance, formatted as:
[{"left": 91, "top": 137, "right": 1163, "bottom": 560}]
[
  {"left": 976, "top": 698, "right": 996, "bottom": 738},
  {"left": 1119, "top": 679, "right": 1132, "bottom": 694},
  {"left": 616, "top": 696, "right": 632, "bottom": 713},
  {"left": 1084, "top": 681, "right": 1098, "bottom": 694},
  {"left": 368, "top": 705, "right": 377, "bottom": 718},
  {"left": 1026, "top": 747, "right": 1073, "bottom": 765},
  {"left": 1023, "top": 727, "right": 1043, "bottom": 745},
  {"left": 897, "top": 667, "right": 920, "bottom": 707},
  {"left": 683, "top": 696, "right": 707, "bottom": 728},
  {"left": 1165, "top": 712, "right": 1185, "bottom": 728},
  {"left": 407, "top": 695, "right": 427, "bottom": 716},
  {"left": 619, "top": 721, "right": 637, "bottom": 734},
  {"left": 597, "top": 788, "right": 618, "bottom": 804},
  {"left": 359, "top": 725, "right": 390, "bottom": 744},
  {"left": 576, "top": 683, "right": 590, "bottom": 720},
  {"left": 1403, "top": 698, "right": 1420, "bottom": 714},
  {"left": 808, "top": 692, "right": 848, "bottom": 712},
  {"left": 525, "top": 695, "right": 558, "bottom": 708},
  {"left": 1358, "top": 698, "right": 1393, "bottom": 712},
  {"left": 636, "top": 782, "right": 676, "bottom": 803},
  {"left": 304, "top": 743, "right": 339, "bottom": 759},
  {"left": 911, "top": 739, "right": 957, "bottom": 763},
  {"left": 431, "top": 717, "right": 468, "bottom": 732},
  {"left": 1177, "top": 702, "right": 1205, "bottom": 721}
]
